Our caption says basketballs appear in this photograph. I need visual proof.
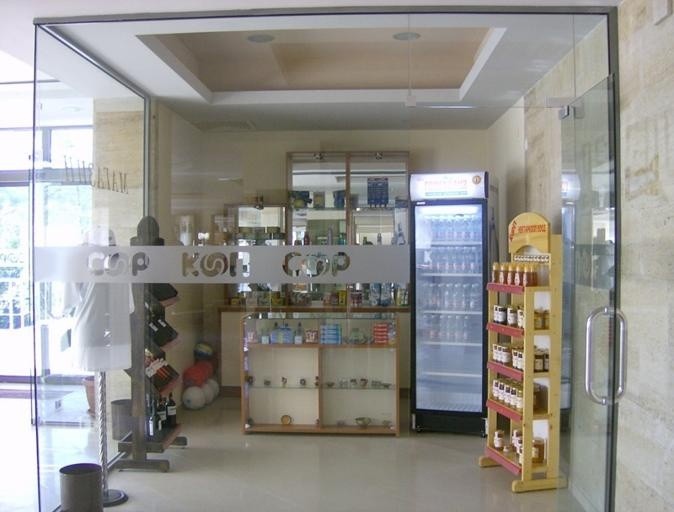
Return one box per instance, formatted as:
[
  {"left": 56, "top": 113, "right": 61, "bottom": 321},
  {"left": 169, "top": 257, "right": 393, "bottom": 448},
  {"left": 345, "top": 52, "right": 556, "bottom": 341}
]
[{"left": 183, "top": 341, "right": 220, "bottom": 410}]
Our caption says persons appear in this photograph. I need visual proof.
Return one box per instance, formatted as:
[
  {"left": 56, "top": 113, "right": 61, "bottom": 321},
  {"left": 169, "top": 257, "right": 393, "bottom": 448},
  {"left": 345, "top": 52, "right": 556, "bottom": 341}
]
[{"left": 59, "top": 225, "right": 133, "bottom": 373}]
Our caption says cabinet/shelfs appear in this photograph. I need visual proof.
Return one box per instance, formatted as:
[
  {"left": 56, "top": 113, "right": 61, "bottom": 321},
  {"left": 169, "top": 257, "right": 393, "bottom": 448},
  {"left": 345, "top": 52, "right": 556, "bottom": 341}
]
[
  {"left": 113, "top": 216, "right": 187, "bottom": 473},
  {"left": 479, "top": 211, "right": 570, "bottom": 494}
]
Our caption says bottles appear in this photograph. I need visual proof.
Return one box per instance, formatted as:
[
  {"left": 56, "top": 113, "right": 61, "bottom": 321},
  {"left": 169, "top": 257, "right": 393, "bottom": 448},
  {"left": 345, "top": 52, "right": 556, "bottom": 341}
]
[
  {"left": 417, "top": 212, "right": 483, "bottom": 343},
  {"left": 294, "top": 189, "right": 347, "bottom": 209},
  {"left": 493, "top": 260, "right": 541, "bottom": 289},
  {"left": 270, "top": 321, "right": 304, "bottom": 344},
  {"left": 530, "top": 437, "right": 546, "bottom": 465},
  {"left": 177, "top": 215, "right": 195, "bottom": 246},
  {"left": 346, "top": 280, "right": 407, "bottom": 307},
  {"left": 140, "top": 301, "right": 177, "bottom": 441},
  {"left": 295, "top": 224, "right": 406, "bottom": 246}
]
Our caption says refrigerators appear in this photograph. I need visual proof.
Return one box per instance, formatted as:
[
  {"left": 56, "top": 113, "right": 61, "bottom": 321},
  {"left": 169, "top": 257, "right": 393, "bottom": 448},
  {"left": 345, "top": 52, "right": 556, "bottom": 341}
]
[{"left": 408, "top": 170, "right": 500, "bottom": 437}]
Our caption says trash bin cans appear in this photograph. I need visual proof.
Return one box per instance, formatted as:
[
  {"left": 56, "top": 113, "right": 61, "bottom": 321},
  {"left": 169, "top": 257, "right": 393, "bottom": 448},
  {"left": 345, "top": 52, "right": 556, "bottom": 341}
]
[
  {"left": 110, "top": 399, "right": 133, "bottom": 440},
  {"left": 59, "top": 463, "right": 103, "bottom": 512}
]
